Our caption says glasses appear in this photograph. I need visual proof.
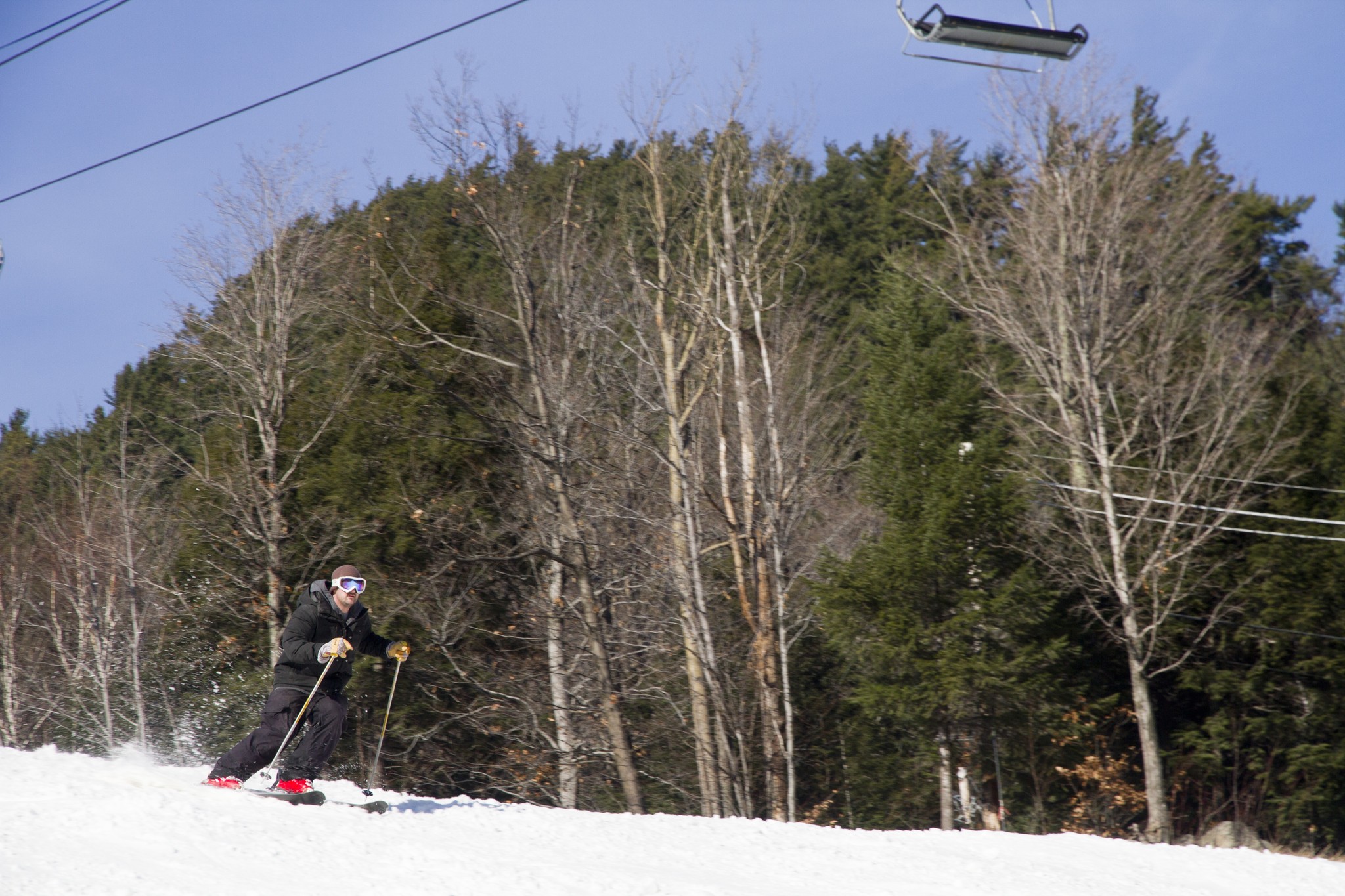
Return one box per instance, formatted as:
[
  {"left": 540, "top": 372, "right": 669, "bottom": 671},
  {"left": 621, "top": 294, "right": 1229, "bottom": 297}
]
[{"left": 338, "top": 577, "right": 366, "bottom": 594}]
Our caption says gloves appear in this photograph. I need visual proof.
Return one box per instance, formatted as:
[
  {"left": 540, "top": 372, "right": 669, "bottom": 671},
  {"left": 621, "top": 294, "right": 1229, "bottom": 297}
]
[
  {"left": 317, "top": 638, "right": 353, "bottom": 663},
  {"left": 386, "top": 640, "right": 411, "bottom": 662}
]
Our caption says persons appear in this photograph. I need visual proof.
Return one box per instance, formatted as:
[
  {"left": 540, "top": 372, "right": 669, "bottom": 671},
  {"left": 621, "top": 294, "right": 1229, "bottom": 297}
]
[{"left": 209, "top": 565, "right": 410, "bottom": 793}]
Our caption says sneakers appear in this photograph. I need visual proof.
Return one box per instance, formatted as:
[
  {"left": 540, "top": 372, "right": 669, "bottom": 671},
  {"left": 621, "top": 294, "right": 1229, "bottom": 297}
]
[
  {"left": 205, "top": 775, "right": 241, "bottom": 789},
  {"left": 276, "top": 776, "right": 314, "bottom": 793}
]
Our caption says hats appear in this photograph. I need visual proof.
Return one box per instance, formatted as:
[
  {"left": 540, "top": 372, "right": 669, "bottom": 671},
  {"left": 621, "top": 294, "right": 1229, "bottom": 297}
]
[{"left": 329, "top": 564, "right": 361, "bottom": 593}]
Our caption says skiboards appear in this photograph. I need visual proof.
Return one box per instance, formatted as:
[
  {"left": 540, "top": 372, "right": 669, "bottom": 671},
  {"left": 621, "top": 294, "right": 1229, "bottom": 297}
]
[{"left": 192, "top": 777, "right": 390, "bottom": 814}]
